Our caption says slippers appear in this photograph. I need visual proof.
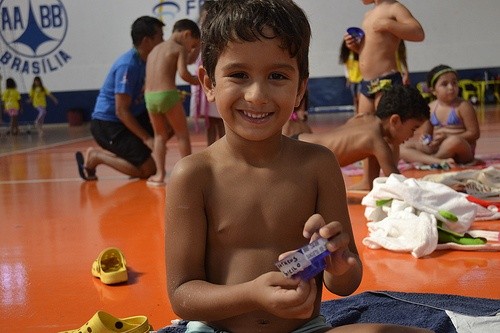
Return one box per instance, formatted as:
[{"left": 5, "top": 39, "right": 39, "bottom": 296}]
[
  {"left": 75, "top": 151, "right": 98, "bottom": 181},
  {"left": 93, "top": 247, "right": 128, "bottom": 284},
  {"left": 57, "top": 310, "right": 150, "bottom": 333},
  {"left": 146, "top": 176, "right": 166, "bottom": 186}
]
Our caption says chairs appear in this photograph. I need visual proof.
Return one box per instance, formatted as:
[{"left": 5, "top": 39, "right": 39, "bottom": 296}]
[{"left": 417, "top": 80, "right": 500, "bottom": 105}]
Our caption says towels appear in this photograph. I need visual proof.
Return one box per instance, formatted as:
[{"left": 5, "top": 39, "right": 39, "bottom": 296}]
[
  {"left": 157, "top": 290, "right": 500, "bottom": 333},
  {"left": 361, "top": 166, "right": 500, "bottom": 260}
]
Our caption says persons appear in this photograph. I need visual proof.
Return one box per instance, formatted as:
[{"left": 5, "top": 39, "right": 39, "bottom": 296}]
[
  {"left": 345, "top": 0, "right": 426, "bottom": 190},
  {"left": 300, "top": 83, "right": 429, "bottom": 176},
  {"left": 163, "top": 0, "right": 438, "bottom": 333},
  {"left": 2, "top": 78, "right": 22, "bottom": 136},
  {"left": 346, "top": 43, "right": 362, "bottom": 115},
  {"left": 144, "top": 18, "right": 201, "bottom": 186},
  {"left": 30, "top": 77, "right": 58, "bottom": 129},
  {"left": 75, "top": 16, "right": 164, "bottom": 180},
  {"left": 399, "top": 64, "right": 480, "bottom": 171}
]
